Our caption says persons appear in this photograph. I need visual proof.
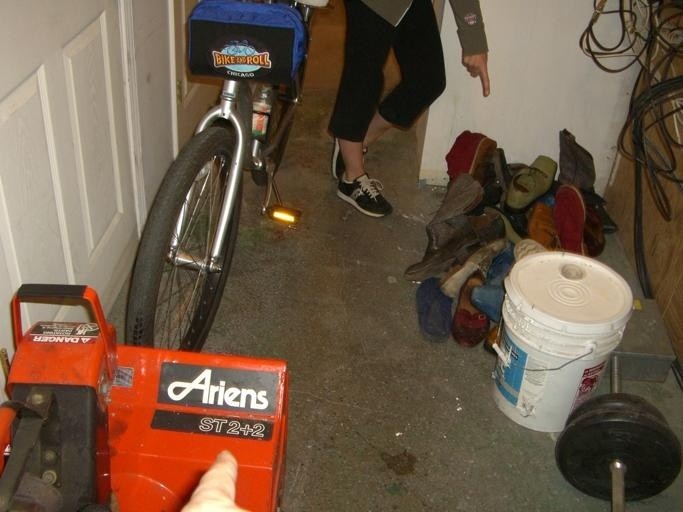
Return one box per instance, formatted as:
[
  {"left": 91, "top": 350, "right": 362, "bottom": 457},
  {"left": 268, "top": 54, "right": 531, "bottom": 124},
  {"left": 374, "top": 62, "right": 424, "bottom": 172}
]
[
  {"left": 178, "top": 449, "right": 247, "bottom": 512},
  {"left": 325, "top": 0, "right": 490, "bottom": 218}
]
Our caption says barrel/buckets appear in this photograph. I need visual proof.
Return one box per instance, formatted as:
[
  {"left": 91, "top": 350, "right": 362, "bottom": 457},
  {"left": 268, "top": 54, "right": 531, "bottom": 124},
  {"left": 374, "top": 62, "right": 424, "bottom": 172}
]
[{"left": 491, "top": 251, "right": 634, "bottom": 432}]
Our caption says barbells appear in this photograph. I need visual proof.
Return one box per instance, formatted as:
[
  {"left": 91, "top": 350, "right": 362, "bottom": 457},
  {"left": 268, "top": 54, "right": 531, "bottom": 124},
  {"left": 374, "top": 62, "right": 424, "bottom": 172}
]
[{"left": 554, "top": 355, "right": 682, "bottom": 512}]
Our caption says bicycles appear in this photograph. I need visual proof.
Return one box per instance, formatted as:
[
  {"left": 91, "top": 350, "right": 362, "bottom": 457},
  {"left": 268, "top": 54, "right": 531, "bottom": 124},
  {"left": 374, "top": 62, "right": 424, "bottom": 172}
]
[{"left": 126, "top": 1, "right": 325, "bottom": 353}]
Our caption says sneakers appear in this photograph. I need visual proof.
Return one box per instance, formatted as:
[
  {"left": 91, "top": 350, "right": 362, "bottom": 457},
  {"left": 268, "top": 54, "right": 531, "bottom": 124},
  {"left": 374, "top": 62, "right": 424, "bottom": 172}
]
[
  {"left": 332, "top": 136, "right": 368, "bottom": 181},
  {"left": 336, "top": 170, "right": 393, "bottom": 217},
  {"left": 404, "top": 129, "right": 618, "bottom": 356}
]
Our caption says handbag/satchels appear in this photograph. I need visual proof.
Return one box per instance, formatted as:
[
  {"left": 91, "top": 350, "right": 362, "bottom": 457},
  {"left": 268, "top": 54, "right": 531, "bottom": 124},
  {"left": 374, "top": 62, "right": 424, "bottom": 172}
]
[{"left": 188, "top": 1, "right": 308, "bottom": 84}]
